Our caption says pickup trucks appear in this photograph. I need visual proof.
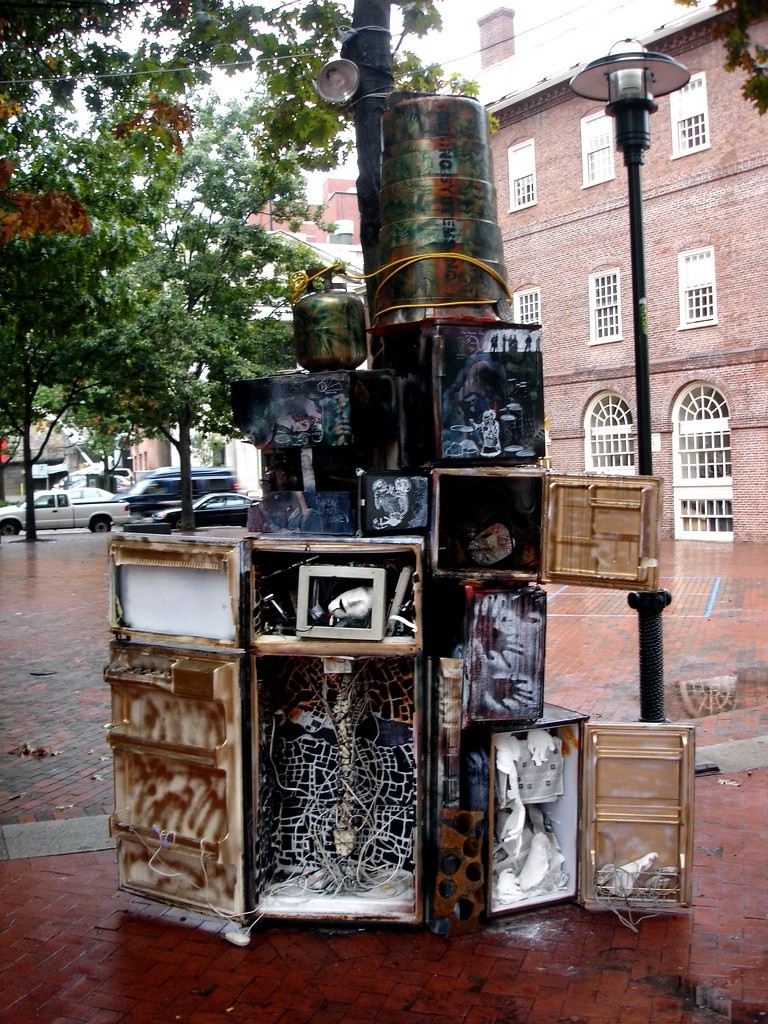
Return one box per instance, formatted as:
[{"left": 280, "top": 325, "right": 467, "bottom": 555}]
[{"left": 0, "top": 487, "right": 131, "bottom": 536}]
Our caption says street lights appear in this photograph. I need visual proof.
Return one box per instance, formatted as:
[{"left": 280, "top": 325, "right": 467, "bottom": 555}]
[{"left": 570, "top": 37, "right": 723, "bottom": 779}]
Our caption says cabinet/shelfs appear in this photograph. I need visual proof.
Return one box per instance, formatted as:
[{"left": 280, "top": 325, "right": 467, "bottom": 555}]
[{"left": 109, "top": 318, "right": 694, "bottom": 932}]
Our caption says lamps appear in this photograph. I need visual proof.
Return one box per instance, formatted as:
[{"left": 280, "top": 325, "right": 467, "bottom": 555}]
[{"left": 317, "top": 58, "right": 360, "bottom": 105}]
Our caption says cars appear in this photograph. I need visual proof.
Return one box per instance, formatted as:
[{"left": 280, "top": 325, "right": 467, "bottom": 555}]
[
  {"left": 52, "top": 468, "right": 136, "bottom": 502},
  {"left": 151, "top": 492, "right": 262, "bottom": 529}
]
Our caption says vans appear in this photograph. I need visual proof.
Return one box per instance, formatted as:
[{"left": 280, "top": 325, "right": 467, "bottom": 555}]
[{"left": 111, "top": 466, "right": 238, "bottom": 503}]
